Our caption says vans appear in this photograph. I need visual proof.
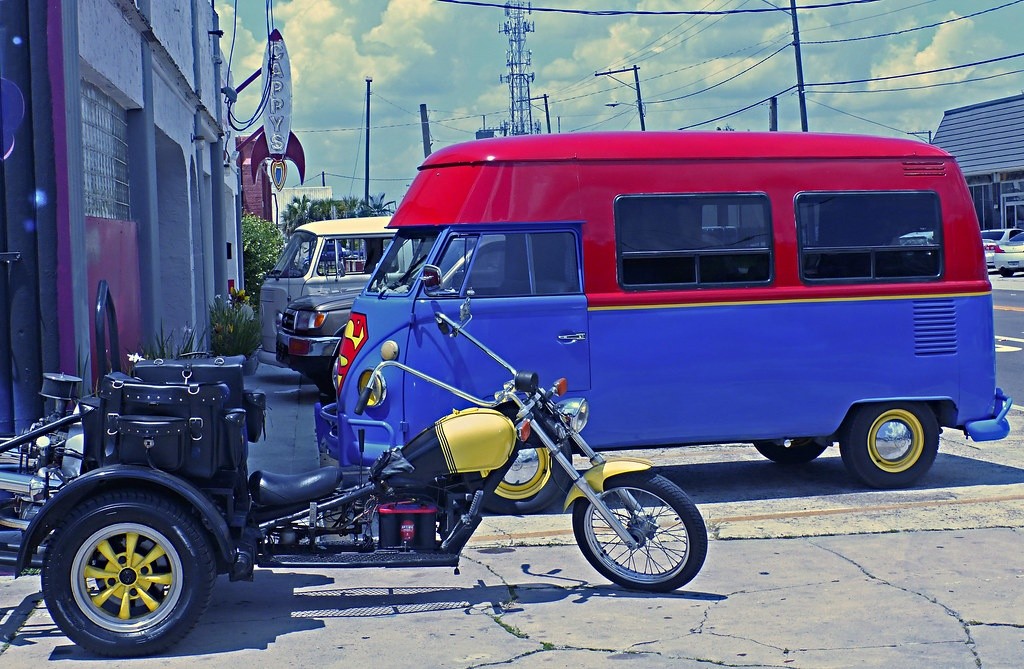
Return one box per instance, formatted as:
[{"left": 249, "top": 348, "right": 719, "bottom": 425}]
[
  {"left": 315, "top": 130, "right": 1012, "bottom": 516},
  {"left": 258, "top": 216, "right": 403, "bottom": 367}
]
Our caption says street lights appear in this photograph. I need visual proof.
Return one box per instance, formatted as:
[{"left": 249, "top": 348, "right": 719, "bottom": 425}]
[{"left": 605, "top": 102, "right": 645, "bottom": 131}]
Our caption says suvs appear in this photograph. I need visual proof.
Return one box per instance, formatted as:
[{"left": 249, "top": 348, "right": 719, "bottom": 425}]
[{"left": 275, "top": 229, "right": 505, "bottom": 399}]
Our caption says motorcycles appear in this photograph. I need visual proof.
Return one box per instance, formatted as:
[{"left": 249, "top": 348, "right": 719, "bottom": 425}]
[{"left": 0, "top": 279, "right": 708, "bottom": 657}]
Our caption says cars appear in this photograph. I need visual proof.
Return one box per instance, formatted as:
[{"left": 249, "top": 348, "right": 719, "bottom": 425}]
[
  {"left": 994, "top": 232, "right": 1024, "bottom": 277},
  {"left": 981, "top": 228, "right": 1024, "bottom": 268}
]
